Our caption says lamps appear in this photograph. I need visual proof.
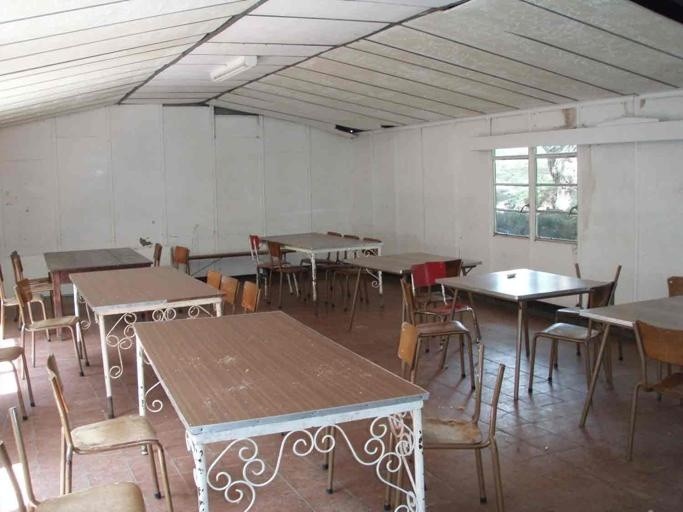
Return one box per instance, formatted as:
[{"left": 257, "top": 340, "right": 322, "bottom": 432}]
[{"left": 210, "top": 54, "right": 257, "bottom": 85}]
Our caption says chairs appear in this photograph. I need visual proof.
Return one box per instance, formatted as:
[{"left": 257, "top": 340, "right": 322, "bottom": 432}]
[
  {"left": 10, "top": 256, "right": 51, "bottom": 343},
  {"left": 665, "top": 276, "right": 683, "bottom": 375},
  {"left": 14, "top": 283, "right": 90, "bottom": 379},
  {"left": 527, "top": 266, "right": 623, "bottom": 407},
  {"left": 240, "top": 280, "right": 262, "bottom": 314},
  {"left": 1, "top": 346, "right": 35, "bottom": 421},
  {"left": 628, "top": 320, "right": 683, "bottom": 454},
  {"left": 0, "top": 408, "right": 147, "bottom": 512},
  {"left": 0, "top": 232, "right": 384, "bottom": 298},
  {"left": 218, "top": 275, "right": 239, "bottom": 314},
  {"left": 325, "top": 321, "right": 424, "bottom": 495},
  {"left": 45, "top": 352, "right": 175, "bottom": 512},
  {"left": 400, "top": 276, "right": 476, "bottom": 391},
  {"left": 409, "top": 264, "right": 455, "bottom": 321},
  {"left": 383, "top": 342, "right": 507, "bottom": 512},
  {"left": 423, "top": 258, "right": 482, "bottom": 350}
]
